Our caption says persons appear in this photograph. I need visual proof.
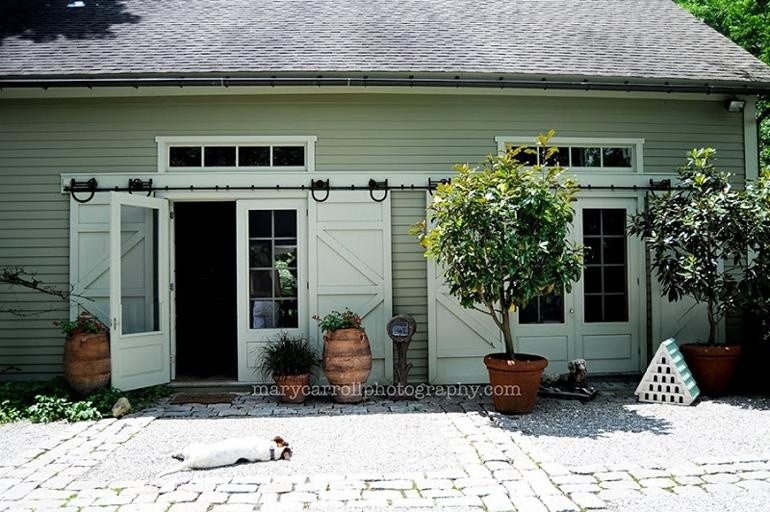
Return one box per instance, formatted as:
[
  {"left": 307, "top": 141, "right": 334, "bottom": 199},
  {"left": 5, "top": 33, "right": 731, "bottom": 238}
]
[{"left": 250, "top": 244, "right": 283, "bottom": 328}]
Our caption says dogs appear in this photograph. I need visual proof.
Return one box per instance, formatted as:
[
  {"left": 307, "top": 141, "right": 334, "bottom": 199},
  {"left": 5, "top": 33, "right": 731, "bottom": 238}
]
[
  {"left": 157, "top": 433, "right": 295, "bottom": 478},
  {"left": 539, "top": 358, "right": 599, "bottom": 399}
]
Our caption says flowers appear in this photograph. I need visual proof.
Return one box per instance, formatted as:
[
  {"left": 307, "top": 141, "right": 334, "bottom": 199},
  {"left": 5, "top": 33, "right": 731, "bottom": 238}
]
[
  {"left": 53, "top": 312, "right": 108, "bottom": 335},
  {"left": 311, "top": 309, "right": 365, "bottom": 331}
]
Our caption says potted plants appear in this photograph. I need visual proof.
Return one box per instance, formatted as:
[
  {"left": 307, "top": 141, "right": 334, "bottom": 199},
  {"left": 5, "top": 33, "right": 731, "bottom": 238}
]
[
  {"left": 409, "top": 129, "right": 595, "bottom": 415},
  {"left": 252, "top": 331, "right": 324, "bottom": 404},
  {"left": 622, "top": 148, "right": 770, "bottom": 399}
]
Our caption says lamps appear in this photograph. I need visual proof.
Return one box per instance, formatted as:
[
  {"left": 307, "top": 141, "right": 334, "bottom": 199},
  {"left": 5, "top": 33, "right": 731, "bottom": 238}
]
[{"left": 723, "top": 96, "right": 747, "bottom": 113}]
